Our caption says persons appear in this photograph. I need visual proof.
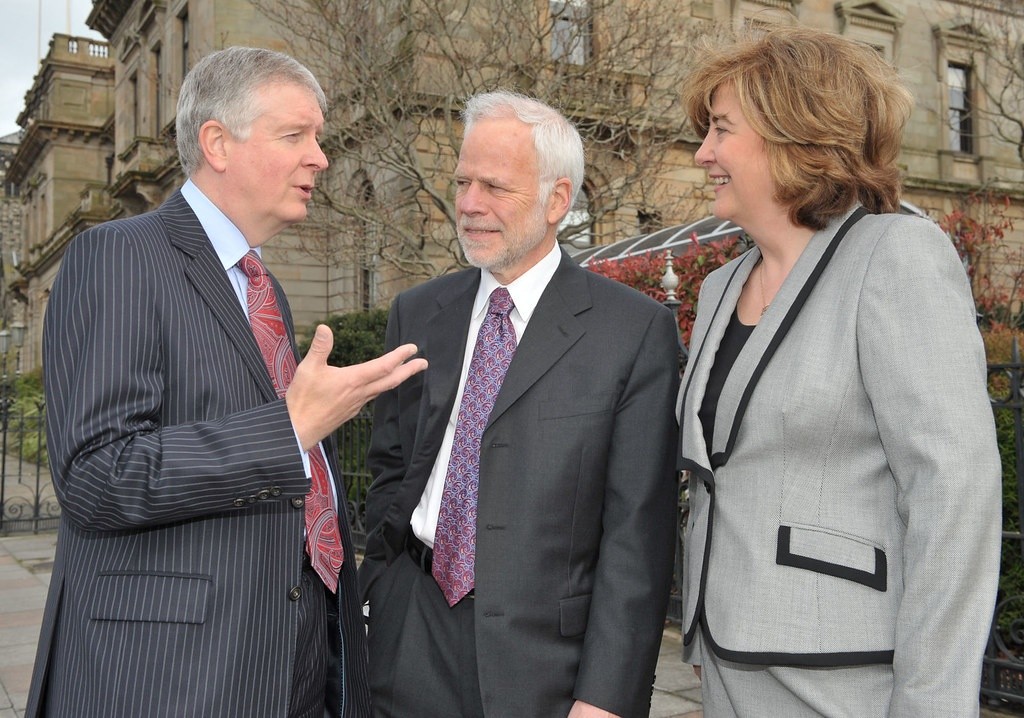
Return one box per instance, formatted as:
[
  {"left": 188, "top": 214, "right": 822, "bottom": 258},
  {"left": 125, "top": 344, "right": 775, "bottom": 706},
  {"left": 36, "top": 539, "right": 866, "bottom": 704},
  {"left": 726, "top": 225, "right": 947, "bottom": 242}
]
[
  {"left": 360, "top": 88, "right": 684, "bottom": 717},
  {"left": 23, "top": 46, "right": 430, "bottom": 718},
  {"left": 671, "top": 30, "right": 1006, "bottom": 718}
]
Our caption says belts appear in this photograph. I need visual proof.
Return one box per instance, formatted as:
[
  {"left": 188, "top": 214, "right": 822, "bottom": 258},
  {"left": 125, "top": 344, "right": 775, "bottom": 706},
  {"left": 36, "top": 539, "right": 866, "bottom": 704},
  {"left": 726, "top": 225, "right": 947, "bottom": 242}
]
[{"left": 408, "top": 525, "right": 476, "bottom": 598}]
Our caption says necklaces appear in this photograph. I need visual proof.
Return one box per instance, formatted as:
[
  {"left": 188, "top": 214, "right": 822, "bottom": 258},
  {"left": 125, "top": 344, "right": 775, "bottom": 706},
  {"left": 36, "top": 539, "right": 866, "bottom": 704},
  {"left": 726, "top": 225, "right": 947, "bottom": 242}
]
[{"left": 756, "top": 258, "right": 773, "bottom": 318}]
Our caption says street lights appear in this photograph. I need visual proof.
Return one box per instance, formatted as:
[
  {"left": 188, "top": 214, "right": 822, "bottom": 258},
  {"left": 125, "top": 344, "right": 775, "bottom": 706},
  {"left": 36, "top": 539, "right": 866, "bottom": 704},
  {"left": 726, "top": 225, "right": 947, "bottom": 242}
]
[
  {"left": 0, "top": 326, "right": 12, "bottom": 397},
  {"left": 9, "top": 319, "right": 27, "bottom": 378}
]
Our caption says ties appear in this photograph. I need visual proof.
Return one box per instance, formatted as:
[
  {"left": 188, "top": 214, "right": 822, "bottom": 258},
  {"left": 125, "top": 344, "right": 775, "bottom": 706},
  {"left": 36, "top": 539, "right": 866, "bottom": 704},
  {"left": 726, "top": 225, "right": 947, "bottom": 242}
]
[
  {"left": 430, "top": 288, "right": 517, "bottom": 607},
  {"left": 236, "top": 251, "right": 344, "bottom": 596}
]
